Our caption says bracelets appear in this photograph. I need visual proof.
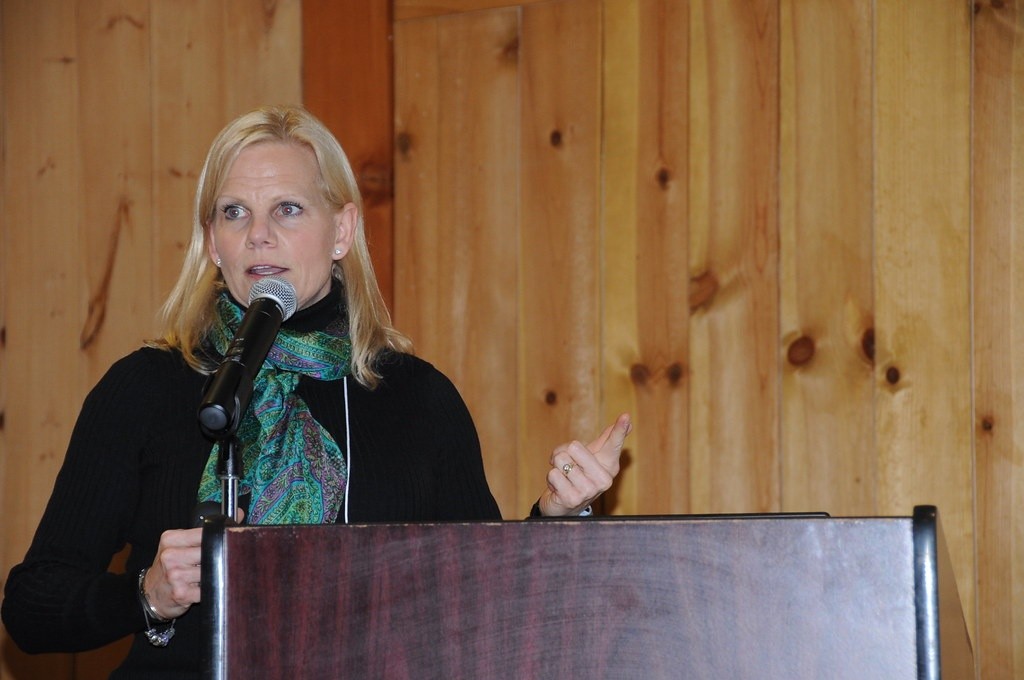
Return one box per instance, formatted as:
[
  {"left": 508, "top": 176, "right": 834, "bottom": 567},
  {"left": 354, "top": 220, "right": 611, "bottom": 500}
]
[{"left": 138, "top": 567, "right": 178, "bottom": 647}]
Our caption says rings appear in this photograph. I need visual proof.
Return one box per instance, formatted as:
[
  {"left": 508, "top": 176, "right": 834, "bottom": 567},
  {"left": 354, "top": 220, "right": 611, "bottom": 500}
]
[{"left": 563, "top": 463, "right": 575, "bottom": 477}]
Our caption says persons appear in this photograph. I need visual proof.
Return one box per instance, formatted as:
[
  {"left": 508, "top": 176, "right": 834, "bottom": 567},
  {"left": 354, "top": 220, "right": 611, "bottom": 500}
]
[{"left": 0, "top": 99, "right": 633, "bottom": 680}]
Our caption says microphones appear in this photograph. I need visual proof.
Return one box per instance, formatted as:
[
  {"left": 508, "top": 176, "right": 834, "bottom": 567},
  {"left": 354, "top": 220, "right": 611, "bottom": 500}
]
[{"left": 196, "top": 275, "right": 298, "bottom": 435}]
